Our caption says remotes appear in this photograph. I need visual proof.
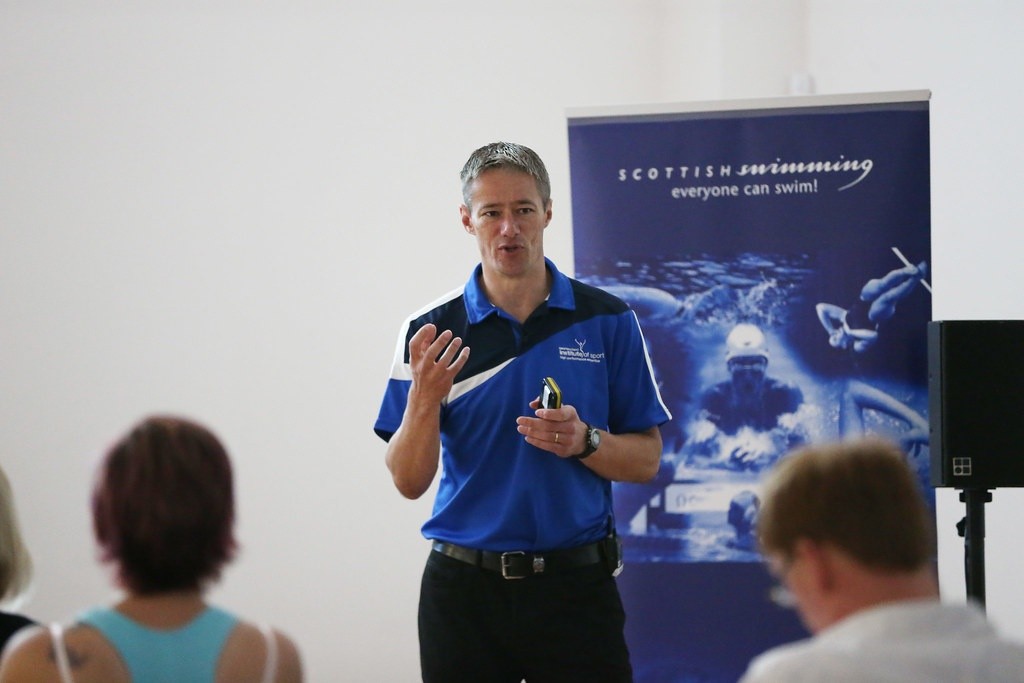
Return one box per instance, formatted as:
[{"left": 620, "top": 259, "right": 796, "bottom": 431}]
[{"left": 538, "top": 377, "right": 561, "bottom": 410}]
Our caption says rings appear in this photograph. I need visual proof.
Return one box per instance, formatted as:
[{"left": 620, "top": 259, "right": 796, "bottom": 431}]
[{"left": 554, "top": 431, "right": 558, "bottom": 442}]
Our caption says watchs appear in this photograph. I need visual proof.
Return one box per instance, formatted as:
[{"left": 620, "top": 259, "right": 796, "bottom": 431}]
[{"left": 573, "top": 420, "right": 601, "bottom": 458}]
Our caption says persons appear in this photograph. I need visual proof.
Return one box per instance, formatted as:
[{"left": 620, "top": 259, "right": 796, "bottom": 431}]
[
  {"left": 738, "top": 437, "right": 1024, "bottom": 683},
  {"left": 687, "top": 323, "right": 808, "bottom": 473},
  {"left": 0, "top": 417, "right": 301, "bottom": 683},
  {"left": 375, "top": 142, "right": 672, "bottom": 683}
]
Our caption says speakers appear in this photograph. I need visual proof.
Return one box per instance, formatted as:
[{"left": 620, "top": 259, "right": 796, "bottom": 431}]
[{"left": 927, "top": 319, "right": 1024, "bottom": 489}]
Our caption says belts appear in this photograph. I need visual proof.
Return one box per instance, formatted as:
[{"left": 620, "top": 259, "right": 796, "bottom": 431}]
[{"left": 432, "top": 532, "right": 615, "bottom": 579}]
